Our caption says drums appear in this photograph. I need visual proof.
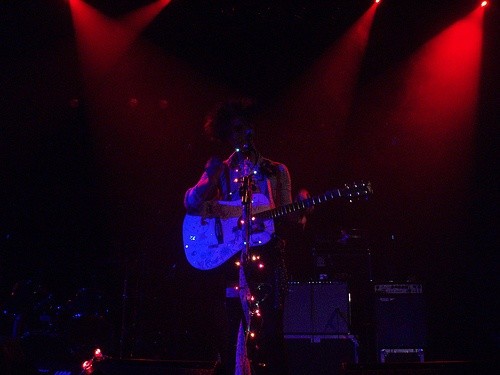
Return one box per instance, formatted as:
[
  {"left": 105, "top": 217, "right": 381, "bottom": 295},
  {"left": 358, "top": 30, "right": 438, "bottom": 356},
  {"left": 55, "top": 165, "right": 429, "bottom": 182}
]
[
  {"left": 3, "top": 279, "right": 55, "bottom": 322},
  {"left": 68, "top": 283, "right": 114, "bottom": 329}
]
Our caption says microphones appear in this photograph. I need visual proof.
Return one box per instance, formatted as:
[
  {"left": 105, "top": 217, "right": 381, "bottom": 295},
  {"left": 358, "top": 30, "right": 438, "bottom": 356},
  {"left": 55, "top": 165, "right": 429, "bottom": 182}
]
[{"left": 243, "top": 135, "right": 249, "bottom": 149}]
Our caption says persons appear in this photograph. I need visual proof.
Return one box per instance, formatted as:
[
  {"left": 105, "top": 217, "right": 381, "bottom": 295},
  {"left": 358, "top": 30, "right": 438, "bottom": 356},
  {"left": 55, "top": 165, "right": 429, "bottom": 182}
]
[
  {"left": 285, "top": 182, "right": 317, "bottom": 281},
  {"left": 184, "top": 99, "right": 298, "bottom": 374}
]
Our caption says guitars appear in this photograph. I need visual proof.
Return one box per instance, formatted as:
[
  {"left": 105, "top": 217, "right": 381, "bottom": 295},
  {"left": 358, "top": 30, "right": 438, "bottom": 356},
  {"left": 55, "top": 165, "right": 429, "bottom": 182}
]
[{"left": 181, "top": 182, "right": 374, "bottom": 270}]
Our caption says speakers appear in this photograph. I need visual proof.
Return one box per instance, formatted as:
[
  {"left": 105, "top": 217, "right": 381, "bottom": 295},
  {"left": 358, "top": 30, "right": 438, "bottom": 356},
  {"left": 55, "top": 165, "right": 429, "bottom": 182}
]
[{"left": 374, "top": 282, "right": 426, "bottom": 362}]
